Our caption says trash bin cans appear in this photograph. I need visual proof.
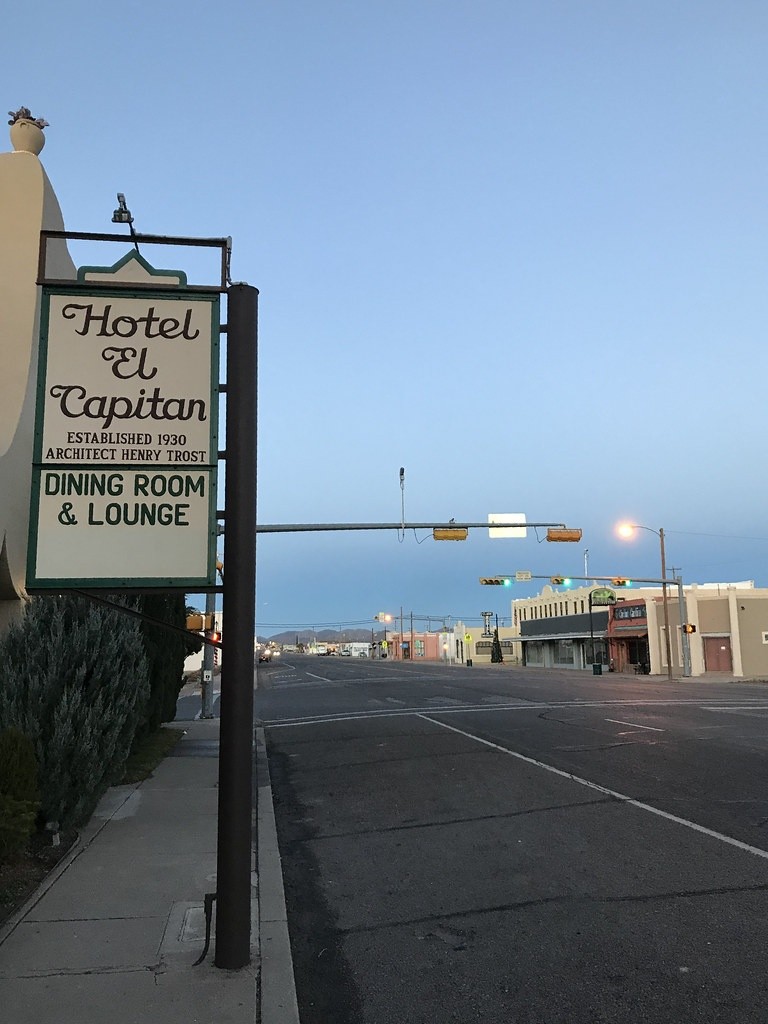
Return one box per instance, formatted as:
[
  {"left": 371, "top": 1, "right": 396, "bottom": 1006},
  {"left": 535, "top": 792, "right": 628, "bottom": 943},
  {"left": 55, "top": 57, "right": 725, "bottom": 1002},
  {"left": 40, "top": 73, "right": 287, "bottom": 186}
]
[
  {"left": 467, "top": 659, "right": 472, "bottom": 667},
  {"left": 593, "top": 663, "right": 602, "bottom": 675}
]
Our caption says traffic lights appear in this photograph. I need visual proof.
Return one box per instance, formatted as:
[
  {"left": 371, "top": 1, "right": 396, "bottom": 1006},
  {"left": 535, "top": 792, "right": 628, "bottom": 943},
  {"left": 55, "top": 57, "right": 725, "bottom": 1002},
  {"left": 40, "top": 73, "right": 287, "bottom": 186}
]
[
  {"left": 552, "top": 578, "right": 569, "bottom": 584},
  {"left": 214, "top": 633, "right": 221, "bottom": 640},
  {"left": 479, "top": 578, "right": 510, "bottom": 586},
  {"left": 684, "top": 624, "right": 696, "bottom": 633},
  {"left": 613, "top": 579, "right": 631, "bottom": 586},
  {"left": 206, "top": 616, "right": 215, "bottom": 630}
]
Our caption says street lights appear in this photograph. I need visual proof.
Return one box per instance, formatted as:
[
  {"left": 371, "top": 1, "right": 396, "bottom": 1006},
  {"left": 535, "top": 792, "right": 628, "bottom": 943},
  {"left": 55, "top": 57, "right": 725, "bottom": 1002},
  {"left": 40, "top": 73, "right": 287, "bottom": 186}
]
[{"left": 621, "top": 525, "right": 673, "bottom": 680}]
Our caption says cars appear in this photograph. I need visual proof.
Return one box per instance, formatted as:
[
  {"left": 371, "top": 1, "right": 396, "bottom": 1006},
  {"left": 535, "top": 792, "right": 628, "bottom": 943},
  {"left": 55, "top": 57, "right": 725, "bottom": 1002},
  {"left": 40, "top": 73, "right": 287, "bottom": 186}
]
[{"left": 358, "top": 652, "right": 367, "bottom": 657}]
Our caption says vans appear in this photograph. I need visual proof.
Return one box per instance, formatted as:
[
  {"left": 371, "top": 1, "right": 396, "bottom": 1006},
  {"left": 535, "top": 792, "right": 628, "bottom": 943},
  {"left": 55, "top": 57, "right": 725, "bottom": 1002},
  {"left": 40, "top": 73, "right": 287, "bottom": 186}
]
[
  {"left": 259, "top": 653, "right": 268, "bottom": 662},
  {"left": 317, "top": 647, "right": 326, "bottom": 656}
]
